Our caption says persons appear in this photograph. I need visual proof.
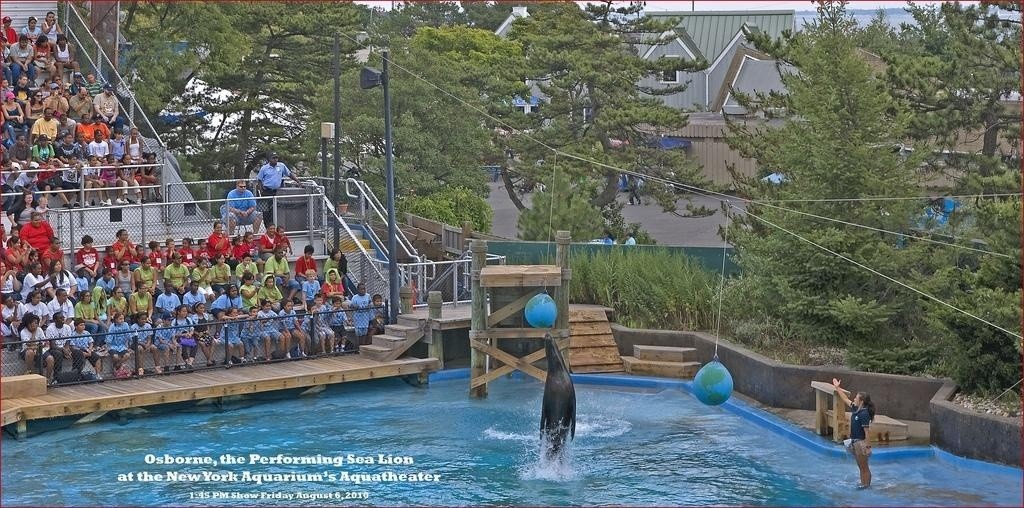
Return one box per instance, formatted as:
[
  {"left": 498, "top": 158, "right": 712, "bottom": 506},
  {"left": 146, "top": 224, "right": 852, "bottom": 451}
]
[
  {"left": 625, "top": 232, "right": 634, "bottom": 245},
  {"left": 832, "top": 378, "right": 875, "bottom": 490},
  {"left": 0, "top": 12, "right": 388, "bottom": 384},
  {"left": 524, "top": 292, "right": 557, "bottom": 328},
  {"left": 628, "top": 185, "right": 644, "bottom": 207},
  {"left": 604, "top": 229, "right": 613, "bottom": 250}
]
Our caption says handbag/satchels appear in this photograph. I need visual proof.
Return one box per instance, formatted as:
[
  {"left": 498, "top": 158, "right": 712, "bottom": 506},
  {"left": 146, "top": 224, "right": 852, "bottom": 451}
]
[{"left": 114, "top": 367, "right": 132, "bottom": 378}]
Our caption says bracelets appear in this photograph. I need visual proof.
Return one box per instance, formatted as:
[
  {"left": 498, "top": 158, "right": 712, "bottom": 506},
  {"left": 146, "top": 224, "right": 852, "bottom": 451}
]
[{"left": 866, "top": 446, "right": 871, "bottom": 448}]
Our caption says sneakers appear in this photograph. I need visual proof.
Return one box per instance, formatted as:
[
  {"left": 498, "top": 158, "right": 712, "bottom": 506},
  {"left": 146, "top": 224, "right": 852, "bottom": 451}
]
[
  {"left": 207, "top": 356, "right": 272, "bottom": 367},
  {"left": 62, "top": 196, "right": 164, "bottom": 209},
  {"left": 95, "top": 373, "right": 103, "bottom": 380},
  {"left": 137, "top": 363, "right": 193, "bottom": 377},
  {"left": 285, "top": 348, "right": 345, "bottom": 358}
]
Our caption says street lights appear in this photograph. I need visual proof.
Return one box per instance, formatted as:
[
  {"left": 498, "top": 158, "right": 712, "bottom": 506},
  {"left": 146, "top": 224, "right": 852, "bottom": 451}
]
[{"left": 360, "top": 52, "right": 399, "bottom": 325}]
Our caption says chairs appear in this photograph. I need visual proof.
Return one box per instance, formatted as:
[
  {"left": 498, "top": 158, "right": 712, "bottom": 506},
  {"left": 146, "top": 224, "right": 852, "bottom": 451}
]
[{"left": 219, "top": 204, "right": 247, "bottom": 235}]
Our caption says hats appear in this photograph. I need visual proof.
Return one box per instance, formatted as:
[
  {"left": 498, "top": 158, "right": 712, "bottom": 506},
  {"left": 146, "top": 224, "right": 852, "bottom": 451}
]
[
  {"left": 50, "top": 83, "right": 61, "bottom": 89},
  {"left": 5, "top": 91, "right": 17, "bottom": 99},
  {"left": 3, "top": 16, "right": 12, "bottom": 23},
  {"left": 74, "top": 72, "right": 82, "bottom": 77},
  {"left": 105, "top": 84, "right": 115, "bottom": 92},
  {"left": 39, "top": 134, "right": 51, "bottom": 141}
]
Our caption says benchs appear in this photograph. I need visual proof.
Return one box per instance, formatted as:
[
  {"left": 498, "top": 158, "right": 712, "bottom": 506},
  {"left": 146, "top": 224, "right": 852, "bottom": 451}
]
[{"left": 0, "top": 1, "right": 58, "bottom": 42}]
